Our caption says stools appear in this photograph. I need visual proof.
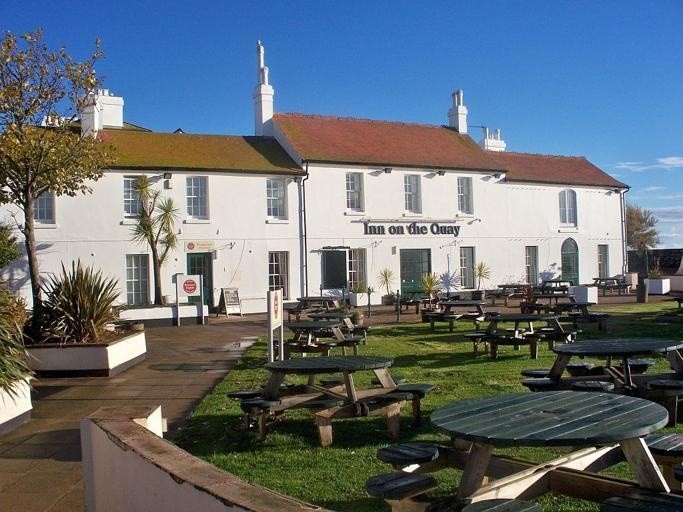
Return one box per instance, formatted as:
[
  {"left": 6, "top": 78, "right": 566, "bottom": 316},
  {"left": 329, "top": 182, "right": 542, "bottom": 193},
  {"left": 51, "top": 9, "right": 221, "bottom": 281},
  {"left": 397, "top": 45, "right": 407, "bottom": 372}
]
[
  {"left": 304, "top": 393, "right": 345, "bottom": 445},
  {"left": 643, "top": 434, "right": 683, "bottom": 491},
  {"left": 226, "top": 386, "right": 259, "bottom": 426},
  {"left": 600, "top": 493, "right": 681, "bottom": 511},
  {"left": 669, "top": 286, "right": 683, "bottom": 317},
  {"left": 376, "top": 391, "right": 410, "bottom": 442},
  {"left": 273, "top": 284, "right": 367, "bottom": 357},
  {"left": 244, "top": 398, "right": 284, "bottom": 442},
  {"left": 398, "top": 381, "right": 435, "bottom": 424},
  {"left": 364, "top": 474, "right": 439, "bottom": 512},
  {"left": 520, "top": 337, "right": 679, "bottom": 427},
  {"left": 378, "top": 442, "right": 442, "bottom": 474},
  {"left": 381, "top": 284, "right": 600, "bottom": 359},
  {"left": 458, "top": 499, "right": 546, "bottom": 512}
]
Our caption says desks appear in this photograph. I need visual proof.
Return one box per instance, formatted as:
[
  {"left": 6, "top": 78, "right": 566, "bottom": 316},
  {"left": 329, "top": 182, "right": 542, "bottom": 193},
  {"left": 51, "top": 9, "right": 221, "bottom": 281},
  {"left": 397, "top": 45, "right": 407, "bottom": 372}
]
[
  {"left": 592, "top": 277, "right": 633, "bottom": 297},
  {"left": 430, "top": 390, "right": 668, "bottom": 493},
  {"left": 262, "top": 356, "right": 398, "bottom": 417}
]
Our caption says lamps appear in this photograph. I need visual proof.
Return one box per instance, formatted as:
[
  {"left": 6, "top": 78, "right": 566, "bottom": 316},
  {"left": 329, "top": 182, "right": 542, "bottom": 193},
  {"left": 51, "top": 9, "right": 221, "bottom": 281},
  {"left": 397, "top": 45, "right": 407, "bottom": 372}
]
[
  {"left": 291, "top": 176, "right": 303, "bottom": 183},
  {"left": 382, "top": 168, "right": 501, "bottom": 178},
  {"left": 612, "top": 188, "right": 621, "bottom": 194},
  {"left": 160, "top": 173, "right": 172, "bottom": 179}
]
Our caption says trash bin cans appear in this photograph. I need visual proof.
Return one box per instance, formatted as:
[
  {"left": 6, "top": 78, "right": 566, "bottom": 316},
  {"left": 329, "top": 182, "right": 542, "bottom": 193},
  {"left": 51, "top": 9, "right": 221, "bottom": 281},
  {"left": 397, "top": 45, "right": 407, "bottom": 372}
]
[
  {"left": 636, "top": 284, "right": 649, "bottom": 303},
  {"left": 625, "top": 271, "right": 639, "bottom": 290}
]
